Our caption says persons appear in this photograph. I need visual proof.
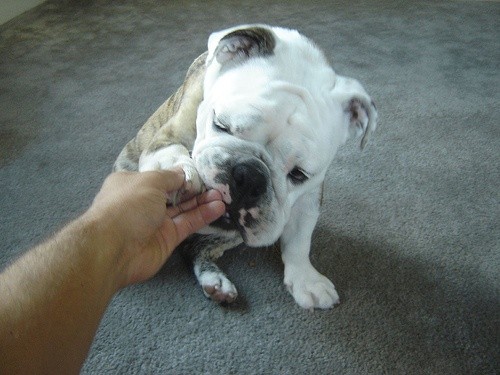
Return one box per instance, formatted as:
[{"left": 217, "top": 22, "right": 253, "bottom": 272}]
[{"left": 0, "top": 168, "right": 225, "bottom": 375}]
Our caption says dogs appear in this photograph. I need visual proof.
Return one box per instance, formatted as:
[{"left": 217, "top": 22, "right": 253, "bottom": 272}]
[{"left": 111, "top": 22, "right": 378, "bottom": 314}]
